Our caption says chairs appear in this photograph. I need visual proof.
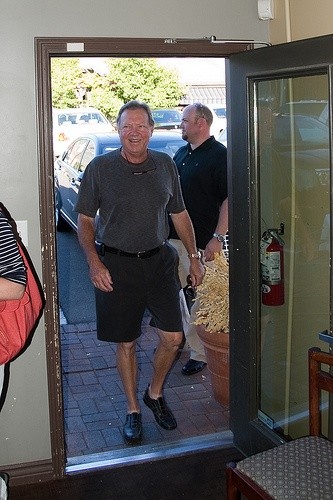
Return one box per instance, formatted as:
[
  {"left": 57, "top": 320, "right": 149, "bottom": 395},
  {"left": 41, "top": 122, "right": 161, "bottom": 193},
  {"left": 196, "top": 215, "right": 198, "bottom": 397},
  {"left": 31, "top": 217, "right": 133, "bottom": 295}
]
[{"left": 226, "top": 347, "right": 333, "bottom": 500}]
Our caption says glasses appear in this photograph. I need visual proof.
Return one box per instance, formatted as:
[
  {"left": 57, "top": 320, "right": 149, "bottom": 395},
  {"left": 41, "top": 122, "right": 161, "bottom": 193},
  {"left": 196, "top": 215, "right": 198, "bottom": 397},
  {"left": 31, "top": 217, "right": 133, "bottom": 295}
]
[
  {"left": 127, "top": 158, "right": 158, "bottom": 176},
  {"left": 186, "top": 274, "right": 197, "bottom": 299}
]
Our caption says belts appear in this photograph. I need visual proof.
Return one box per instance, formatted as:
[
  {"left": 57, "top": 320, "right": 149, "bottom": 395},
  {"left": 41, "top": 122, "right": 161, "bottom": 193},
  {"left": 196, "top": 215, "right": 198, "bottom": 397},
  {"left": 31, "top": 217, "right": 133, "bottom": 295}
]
[{"left": 102, "top": 240, "right": 167, "bottom": 260}]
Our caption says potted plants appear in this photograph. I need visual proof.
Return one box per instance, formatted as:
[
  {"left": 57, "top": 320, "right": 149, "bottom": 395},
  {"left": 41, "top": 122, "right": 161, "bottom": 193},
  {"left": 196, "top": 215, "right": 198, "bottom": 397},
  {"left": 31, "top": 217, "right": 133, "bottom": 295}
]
[{"left": 190, "top": 250, "right": 230, "bottom": 409}]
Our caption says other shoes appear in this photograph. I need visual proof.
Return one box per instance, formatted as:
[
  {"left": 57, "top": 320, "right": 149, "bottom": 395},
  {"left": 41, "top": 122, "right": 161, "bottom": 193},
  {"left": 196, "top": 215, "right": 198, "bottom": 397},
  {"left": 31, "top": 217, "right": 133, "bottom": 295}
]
[{"left": 182, "top": 359, "right": 207, "bottom": 375}]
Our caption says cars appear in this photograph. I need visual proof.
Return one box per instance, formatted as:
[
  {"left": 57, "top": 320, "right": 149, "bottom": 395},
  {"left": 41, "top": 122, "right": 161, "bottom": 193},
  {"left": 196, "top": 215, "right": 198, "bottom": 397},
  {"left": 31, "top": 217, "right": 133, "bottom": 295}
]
[
  {"left": 151, "top": 109, "right": 182, "bottom": 128},
  {"left": 207, "top": 104, "right": 227, "bottom": 139},
  {"left": 54, "top": 131, "right": 188, "bottom": 233},
  {"left": 274, "top": 114, "right": 329, "bottom": 183},
  {"left": 52, "top": 108, "right": 119, "bottom": 156}
]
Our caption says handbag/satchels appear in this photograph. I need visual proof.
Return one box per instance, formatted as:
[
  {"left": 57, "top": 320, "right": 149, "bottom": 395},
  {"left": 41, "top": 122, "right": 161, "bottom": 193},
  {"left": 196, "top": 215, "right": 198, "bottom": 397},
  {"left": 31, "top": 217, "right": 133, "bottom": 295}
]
[{"left": 0, "top": 240, "right": 42, "bottom": 366}]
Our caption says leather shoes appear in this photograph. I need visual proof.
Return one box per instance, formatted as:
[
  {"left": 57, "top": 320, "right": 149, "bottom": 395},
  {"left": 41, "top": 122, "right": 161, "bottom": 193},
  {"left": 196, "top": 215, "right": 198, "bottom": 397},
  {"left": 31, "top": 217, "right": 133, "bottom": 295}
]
[
  {"left": 143, "top": 384, "right": 177, "bottom": 430},
  {"left": 123, "top": 412, "right": 142, "bottom": 443}
]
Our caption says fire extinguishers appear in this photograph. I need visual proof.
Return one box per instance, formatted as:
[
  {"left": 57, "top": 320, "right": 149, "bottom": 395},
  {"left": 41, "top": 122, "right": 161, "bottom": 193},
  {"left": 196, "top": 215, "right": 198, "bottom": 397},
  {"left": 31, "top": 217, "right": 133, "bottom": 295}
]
[{"left": 260, "top": 228, "right": 285, "bottom": 306}]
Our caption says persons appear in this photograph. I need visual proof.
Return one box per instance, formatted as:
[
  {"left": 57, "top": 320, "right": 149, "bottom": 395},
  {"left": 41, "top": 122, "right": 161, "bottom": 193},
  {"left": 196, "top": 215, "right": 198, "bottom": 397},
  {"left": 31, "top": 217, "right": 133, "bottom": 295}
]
[
  {"left": 0, "top": 201, "right": 45, "bottom": 367},
  {"left": 172, "top": 102, "right": 227, "bottom": 263},
  {"left": 76, "top": 101, "right": 206, "bottom": 446}
]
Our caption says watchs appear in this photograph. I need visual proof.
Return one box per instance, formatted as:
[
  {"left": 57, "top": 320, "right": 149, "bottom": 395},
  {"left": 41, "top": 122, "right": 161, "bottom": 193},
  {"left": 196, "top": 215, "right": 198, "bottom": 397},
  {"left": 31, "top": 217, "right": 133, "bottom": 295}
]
[
  {"left": 212, "top": 232, "right": 226, "bottom": 243},
  {"left": 188, "top": 251, "right": 202, "bottom": 259}
]
